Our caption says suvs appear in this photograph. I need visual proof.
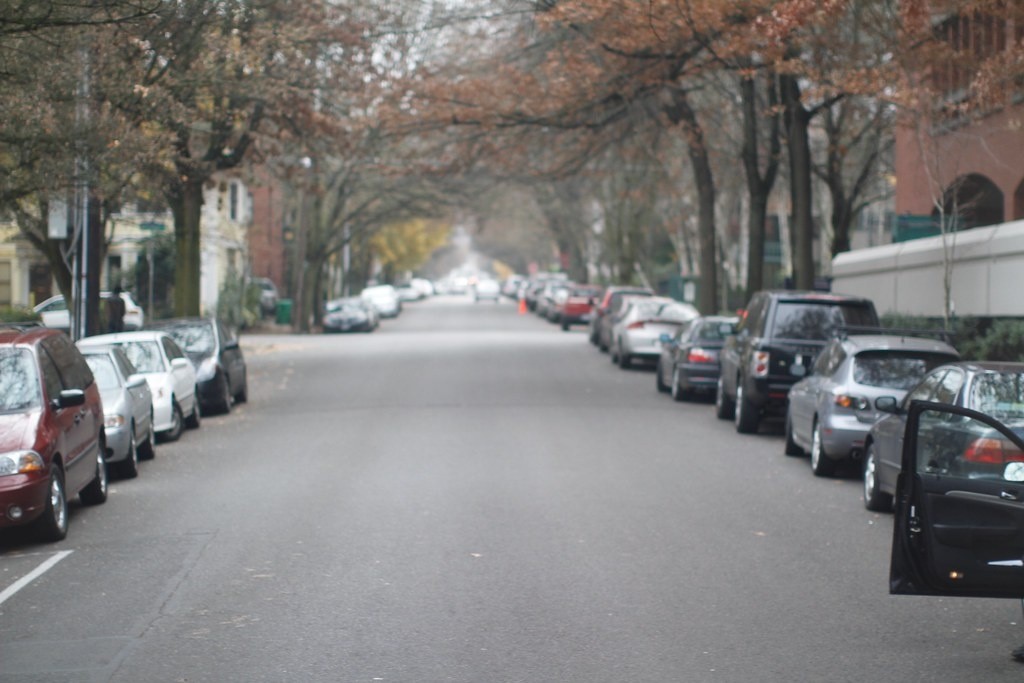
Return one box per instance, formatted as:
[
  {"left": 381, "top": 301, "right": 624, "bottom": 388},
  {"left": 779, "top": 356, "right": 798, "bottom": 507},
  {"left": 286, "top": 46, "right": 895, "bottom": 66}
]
[
  {"left": 0, "top": 323, "right": 108, "bottom": 542},
  {"left": 716, "top": 289, "right": 883, "bottom": 434},
  {"left": 249, "top": 277, "right": 278, "bottom": 314},
  {"left": 31, "top": 292, "right": 143, "bottom": 335}
]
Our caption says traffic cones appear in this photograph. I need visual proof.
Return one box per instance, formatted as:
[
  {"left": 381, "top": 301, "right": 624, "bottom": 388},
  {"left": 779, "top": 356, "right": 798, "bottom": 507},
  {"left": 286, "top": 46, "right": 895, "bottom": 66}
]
[{"left": 517, "top": 298, "right": 527, "bottom": 313}]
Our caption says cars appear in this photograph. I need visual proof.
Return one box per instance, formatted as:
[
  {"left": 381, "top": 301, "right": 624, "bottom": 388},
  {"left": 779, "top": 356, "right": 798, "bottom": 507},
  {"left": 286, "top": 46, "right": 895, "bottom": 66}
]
[
  {"left": 476, "top": 281, "right": 501, "bottom": 301},
  {"left": 139, "top": 319, "right": 248, "bottom": 417},
  {"left": 75, "top": 332, "right": 203, "bottom": 441},
  {"left": 889, "top": 401, "right": 1024, "bottom": 601},
  {"left": 783, "top": 335, "right": 963, "bottom": 476},
  {"left": 861, "top": 358, "right": 1024, "bottom": 514},
  {"left": 399, "top": 278, "right": 468, "bottom": 300},
  {"left": 361, "top": 284, "right": 402, "bottom": 318},
  {"left": 656, "top": 316, "right": 739, "bottom": 401},
  {"left": 503, "top": 272, "right": 702, "bottom": 369},
  {"left": 322, "top": 293, "right": 379, "bottom": 332},
  {"left": 74, "top": 344, "right": 155, "bottom": 479}
]
